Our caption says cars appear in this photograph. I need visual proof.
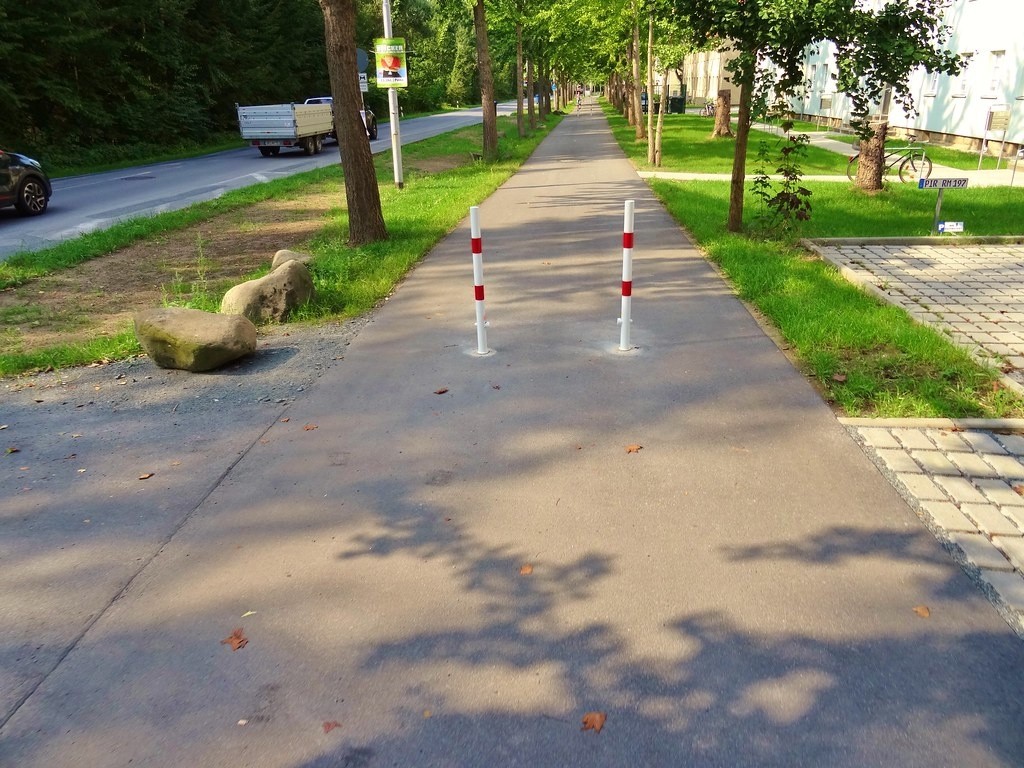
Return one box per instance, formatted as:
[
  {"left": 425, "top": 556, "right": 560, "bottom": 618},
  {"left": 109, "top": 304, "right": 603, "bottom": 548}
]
[
  {"left": 641, "top": 92, "right": 655, "bottom": 112},
  {"left": 0, "top": 148, "right": 54, "bottom": 217}
]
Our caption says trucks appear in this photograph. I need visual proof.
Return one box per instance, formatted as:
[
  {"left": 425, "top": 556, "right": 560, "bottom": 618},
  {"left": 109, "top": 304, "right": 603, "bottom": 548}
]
[{"left": 234, "top": 96, "right": 379, "bottom": 158}]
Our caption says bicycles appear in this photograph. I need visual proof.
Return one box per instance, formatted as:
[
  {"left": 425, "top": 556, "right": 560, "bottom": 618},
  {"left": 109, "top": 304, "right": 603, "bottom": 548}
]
[
  {"left": 846, "top": 133, "right": 932, "bottom": 184},
  {"left": 699, "top": 101, "right": 717, "bottom": 118}
]
